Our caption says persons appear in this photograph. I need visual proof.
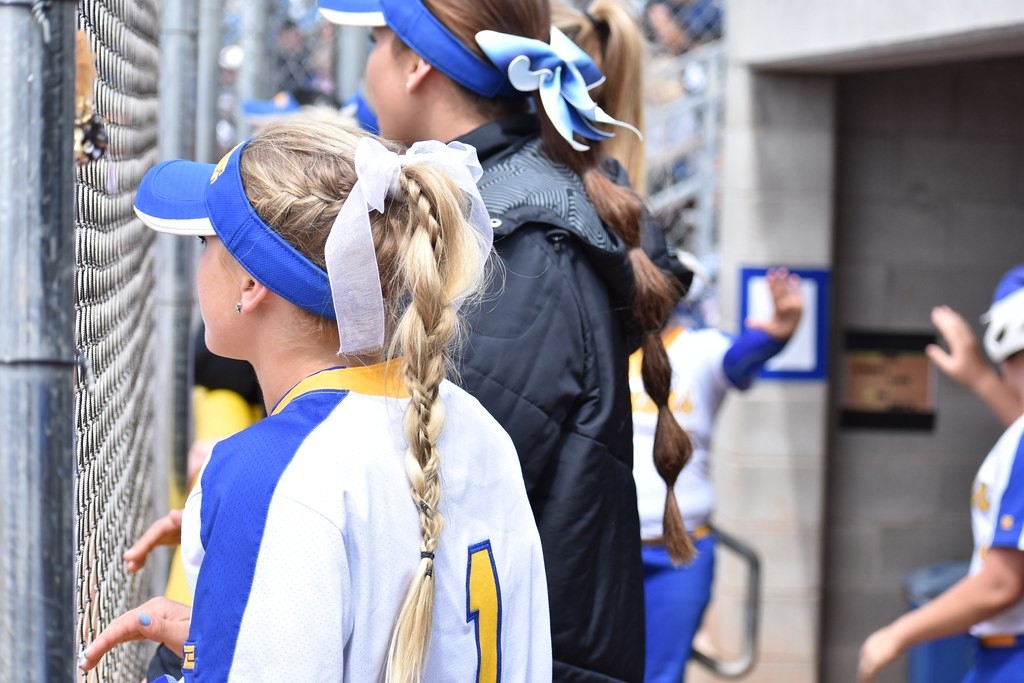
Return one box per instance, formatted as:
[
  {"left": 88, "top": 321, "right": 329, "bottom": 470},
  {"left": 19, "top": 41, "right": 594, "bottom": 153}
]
[
  {"left": 147, "top": 0, "right": 724, "bottom": 683},
  {"left": 79, "top": 117, "right": 552, "bottom": 683},
  {"left": 856, "top": 264, "right": 1024, "bottom": 683},
  {"left": 630, "top": 267, "right": 802, "bottom": 683}
]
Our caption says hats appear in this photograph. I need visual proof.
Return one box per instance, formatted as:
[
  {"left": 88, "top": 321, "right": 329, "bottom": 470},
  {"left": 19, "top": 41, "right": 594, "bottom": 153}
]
[
  {"left": 978, "top": 266, "right": 1024, "bottom": 366},
  {"left": 317, "top": 1, "right": 508, "bottom": 103},
  {"left": 131, "top": 133, "right": 338, "bottom": 325}
]
